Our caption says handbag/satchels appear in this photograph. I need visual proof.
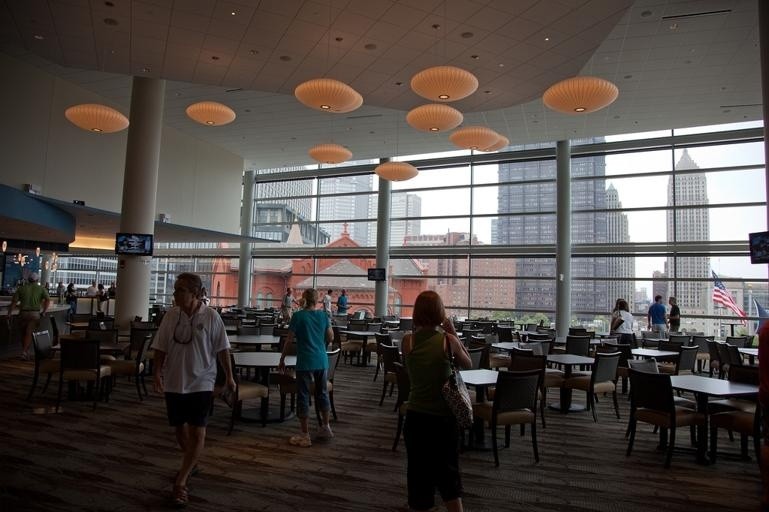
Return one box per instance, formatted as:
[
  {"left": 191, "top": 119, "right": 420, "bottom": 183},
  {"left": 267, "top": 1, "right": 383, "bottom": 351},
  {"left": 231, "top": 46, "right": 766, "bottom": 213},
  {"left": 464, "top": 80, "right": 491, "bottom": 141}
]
[
  {"left": 442, "top": 369, "right": 473, "bottom": 428},
  {"left": 611, "top": 316, "right": 624, "bottom": 331}
]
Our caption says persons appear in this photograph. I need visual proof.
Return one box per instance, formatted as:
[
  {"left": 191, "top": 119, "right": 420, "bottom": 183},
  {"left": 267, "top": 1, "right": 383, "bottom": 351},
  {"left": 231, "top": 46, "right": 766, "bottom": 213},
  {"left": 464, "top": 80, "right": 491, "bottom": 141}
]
[
  {"left": 610, "top": 298, "right": 625, "bottom": 332},
  {"left": 277, "top": 288, "right": 336, "bottom": 448},
  {"left": 610, "top": 300, "right": 635, "bottom": 367},
  {"left": 666, "top": 297, "right": 680, "bottom": 332},
  {"left": 399, "top": 290, "right": 473, "bottom": 511},
  {"left": 647, "top": 294, "right": 669, "bottom": 332},
  {"left": 5, "top": 272, "right": 50, "bottom": 361},
  {"left": 336, "top": 288, "right": 352, "bottom": 314},
  {"left": 15, "top": 277, "right": 116, "bottom": 324},
  {"left": 281, "top": 287, "right": 300, "bottom": 325},
  {"left": 149, "top": 272, "right": 238, "bottom": 508},
  {"left": 323, "top": 289, "right": 333, "bottom": 318}
]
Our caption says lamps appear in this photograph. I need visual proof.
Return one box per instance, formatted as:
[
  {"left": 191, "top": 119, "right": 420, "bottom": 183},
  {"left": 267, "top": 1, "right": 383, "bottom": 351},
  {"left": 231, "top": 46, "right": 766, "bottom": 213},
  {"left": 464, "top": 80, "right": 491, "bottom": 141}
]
[
  {"left": 63, "top": 103, "right": 130, "bottom": 134},
  {"left": 409, "top": 65, "right": 479, "bottom": 103},
  {"left": 541, "top": 76, "right": 620, "bottom": 117},
  {"left": 185, "top": 100, "right": 237, "bottom": 127},
  {"left": 374, "top": 162, "right": 419, "bottom": 181},
  {"left": 294, "top": 78, "right": 364, "bottom": 114},
  {"left": 405, "top": 103, "right": 465, "bottom": 134},
  {"left": 2, "top": 239, "right": 59, "bottom": 274},
  {"left": 447, "top": 125, "right": 509, "bottom": 154},
  {"left": 307, "top": 143, "right": 352, "bottom": 164}
]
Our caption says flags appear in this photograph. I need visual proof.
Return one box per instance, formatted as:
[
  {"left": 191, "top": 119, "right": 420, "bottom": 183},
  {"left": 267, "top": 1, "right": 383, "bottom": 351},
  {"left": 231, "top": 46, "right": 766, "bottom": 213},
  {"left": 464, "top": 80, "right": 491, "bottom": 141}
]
[
  {"left": 752, "top": 298, "right": 769, "bottom": 335},
  {"left": 711, "top": 269, "right": 748, "bottom": 329}
]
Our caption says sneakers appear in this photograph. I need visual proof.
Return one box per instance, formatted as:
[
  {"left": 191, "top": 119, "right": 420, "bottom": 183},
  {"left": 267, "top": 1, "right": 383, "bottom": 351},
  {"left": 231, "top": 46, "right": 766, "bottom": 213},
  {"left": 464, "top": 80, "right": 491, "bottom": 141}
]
[
  {"left": 290, "top": 434, "right": 311, "bottom": 447},
  {"left": 313, "top": 425, "right": 335, "bottom": 438},
  {"left": 17, "top": 352, "right": 28, "bottom": 359}
]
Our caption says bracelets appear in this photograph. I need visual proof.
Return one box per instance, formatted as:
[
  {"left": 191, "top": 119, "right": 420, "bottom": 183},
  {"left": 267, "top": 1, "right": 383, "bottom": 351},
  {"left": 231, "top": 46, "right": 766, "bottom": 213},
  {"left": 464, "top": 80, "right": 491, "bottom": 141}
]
[{"left": 280, "top": 357, "right": 284, "bottom": 362}]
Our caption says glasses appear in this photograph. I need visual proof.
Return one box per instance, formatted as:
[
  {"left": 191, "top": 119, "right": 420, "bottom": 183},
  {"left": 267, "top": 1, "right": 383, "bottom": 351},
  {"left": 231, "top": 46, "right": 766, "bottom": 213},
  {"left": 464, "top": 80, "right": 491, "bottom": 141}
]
[{"left": 173, "top": 323, "right": 193, "bottom": 344}]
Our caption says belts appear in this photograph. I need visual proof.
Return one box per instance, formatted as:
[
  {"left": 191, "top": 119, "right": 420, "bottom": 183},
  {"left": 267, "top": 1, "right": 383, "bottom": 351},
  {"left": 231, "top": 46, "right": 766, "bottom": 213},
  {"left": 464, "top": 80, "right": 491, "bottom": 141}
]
[{"left": 21, "top": 309, "right": 40, "bottom": 312}]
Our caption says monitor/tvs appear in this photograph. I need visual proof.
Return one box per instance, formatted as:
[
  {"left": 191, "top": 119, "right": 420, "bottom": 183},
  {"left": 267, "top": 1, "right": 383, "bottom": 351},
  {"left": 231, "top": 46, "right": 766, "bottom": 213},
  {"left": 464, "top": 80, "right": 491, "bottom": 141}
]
[
  {"left": 368, "top": 268, "right": 385, "bottom": 281},
  {"left": 749, "top": 230, "right": 769, "bottom": 264},
  {"left": 115, "top": 233, "right": 153, "bottom": 256}
]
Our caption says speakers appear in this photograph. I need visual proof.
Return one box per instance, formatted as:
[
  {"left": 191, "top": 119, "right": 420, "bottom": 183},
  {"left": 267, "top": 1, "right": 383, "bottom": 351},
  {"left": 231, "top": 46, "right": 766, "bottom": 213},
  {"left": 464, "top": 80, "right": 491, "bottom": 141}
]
[
  {"left": 23, "top": 183, "right": 41, "bottom": 196},
  {"left": 160, "top": 214, "right": 171, "bottom": 223}
]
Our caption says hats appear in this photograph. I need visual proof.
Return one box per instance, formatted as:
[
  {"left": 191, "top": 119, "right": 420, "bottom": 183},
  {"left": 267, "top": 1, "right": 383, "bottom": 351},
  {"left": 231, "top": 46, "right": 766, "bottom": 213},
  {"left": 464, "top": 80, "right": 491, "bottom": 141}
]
[{"left": 28, "top": 272, "right": 40, "bottom": 282}]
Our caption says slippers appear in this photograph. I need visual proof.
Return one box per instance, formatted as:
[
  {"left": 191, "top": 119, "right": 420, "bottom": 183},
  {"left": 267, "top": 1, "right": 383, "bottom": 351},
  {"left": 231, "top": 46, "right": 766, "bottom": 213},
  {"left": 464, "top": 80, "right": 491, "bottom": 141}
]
[{"left": 172, "top": 474, "right": 189, "bottom": 507}]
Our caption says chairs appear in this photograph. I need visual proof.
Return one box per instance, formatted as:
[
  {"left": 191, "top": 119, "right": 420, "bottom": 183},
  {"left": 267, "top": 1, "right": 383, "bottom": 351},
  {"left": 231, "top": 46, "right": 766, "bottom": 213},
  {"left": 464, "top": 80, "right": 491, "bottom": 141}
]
[{"left": 27, "top": 303, "right": 769, "bottom": 468}]
